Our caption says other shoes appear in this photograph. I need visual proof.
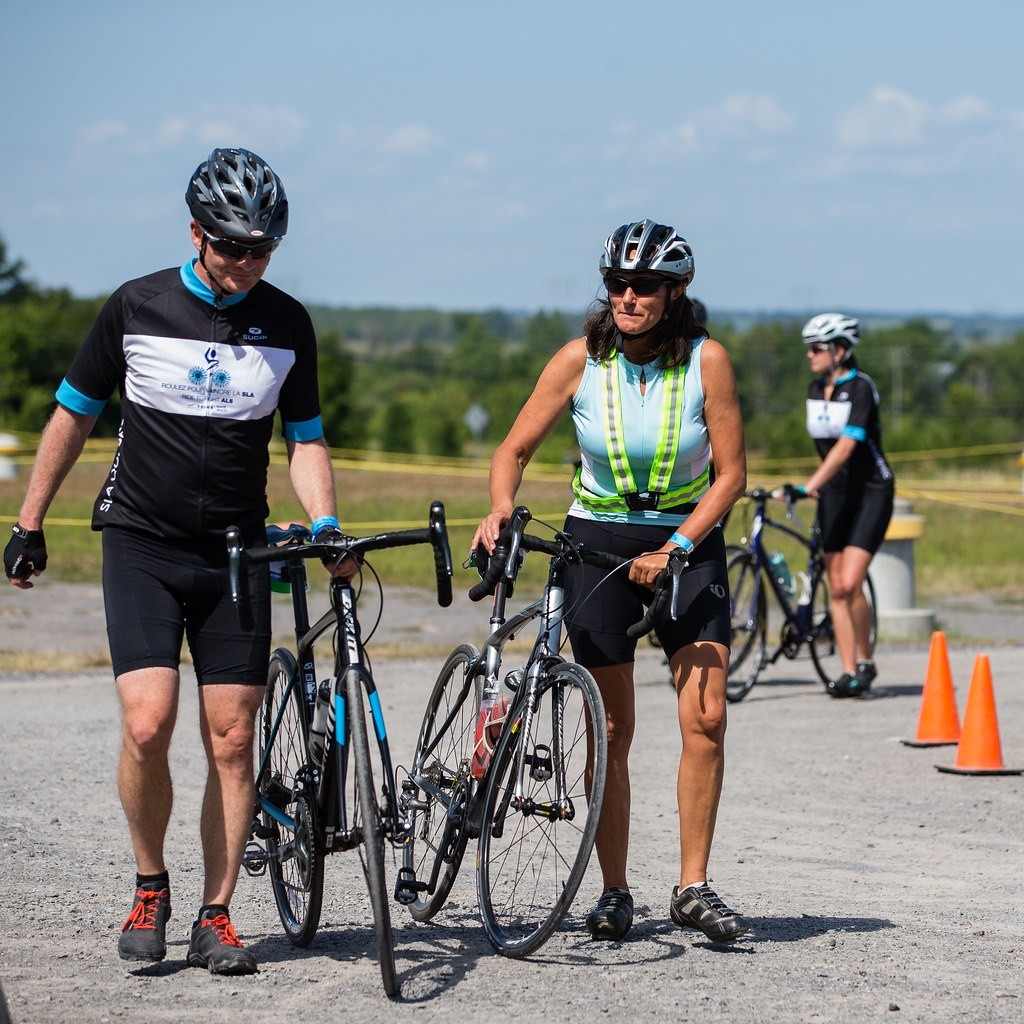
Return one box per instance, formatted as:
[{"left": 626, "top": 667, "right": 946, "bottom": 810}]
[
  {"left": 829, "top": 673, "right": 864, "bottom": 696},
  {"left": 857, "top": 663, "right": 877, "bottom": 680}
]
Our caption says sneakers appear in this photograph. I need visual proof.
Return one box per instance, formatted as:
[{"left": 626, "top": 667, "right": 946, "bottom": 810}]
[
  {"left": 670, "top": 884, "right": 748, "bottom": 940},
  {"left": 187, "top": 909, "right": 257, "bottom": 973},
  {"left": 118, "top": 886, "right": 171, "bottom": 962},
  {"left": 591, "top": 888, "right": 632, "bottom": 936}
]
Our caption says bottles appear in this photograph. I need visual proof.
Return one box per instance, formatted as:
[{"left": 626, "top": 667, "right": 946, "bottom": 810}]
[
  {"left": 770, "top": 550, "right": 794, "bottom": 597},
  {"left": 471, "top": 681, "right": 501, "bottom": 778},
  {"left": 490, "top": 665, "right": 525, "bottom": 745},
  {"left": 309, "top": 677, "right": 332, "bottom": 766}
]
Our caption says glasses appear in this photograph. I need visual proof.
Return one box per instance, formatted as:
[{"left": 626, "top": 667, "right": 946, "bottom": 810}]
[
  {"left": 196, "top": 224, "right": 282, "bottom": 259},
  {"left": 809, "top": 342, "right": 838, "bottom": 353},
  {"left": 601, "top": 271, "right": 679, "bottom": 298}
]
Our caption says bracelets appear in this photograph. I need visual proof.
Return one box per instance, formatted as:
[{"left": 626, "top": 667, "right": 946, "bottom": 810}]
[
  {"left": 793, "top": 485, "right": 805, "bottom": 496},
  {"left": 669, "top": 532, "right": 695, "bottom": 556},
  {"left": 309, "top": 515, "right": 340, "bottom": 534}
]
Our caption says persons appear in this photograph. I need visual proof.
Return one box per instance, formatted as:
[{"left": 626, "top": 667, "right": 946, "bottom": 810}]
[
  {"left": 3, "top": 143, "right": 362, "bottom": 976},
  {"left": 472, "top": 220, "right": 756, "bottom": 941},
  {"left": 771, "top": 310, "right": 896, "bottom": 695}
]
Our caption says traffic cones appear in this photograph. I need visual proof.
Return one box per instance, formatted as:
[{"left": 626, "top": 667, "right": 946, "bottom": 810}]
[
  {"left": 932, "top": 655, "right": 1023, "bottom": 775},
  {"left": 900, "top": 631, "right": 961, "bottom": 746}
]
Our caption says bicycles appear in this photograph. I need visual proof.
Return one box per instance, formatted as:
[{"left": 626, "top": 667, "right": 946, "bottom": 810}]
[
  {"left": 225, "top": 500, "right": 455, "bottom": 996},
  {"left": 403, "top": 505, "right": 687, "bottom": 959},
  {"left": 725, "top": 489, "right": 877, "bottom": 702}
]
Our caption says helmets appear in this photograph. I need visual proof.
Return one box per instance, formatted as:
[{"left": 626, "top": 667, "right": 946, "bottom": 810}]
[
  {"left": 600, "top": 219, "right": 694, "bottom": 284},
  {"left": 802, "top": 312, "right": 860, "bottom": 345},
  {"left": 186, "top": 147, "right": 289, "bottom": 236}
]
